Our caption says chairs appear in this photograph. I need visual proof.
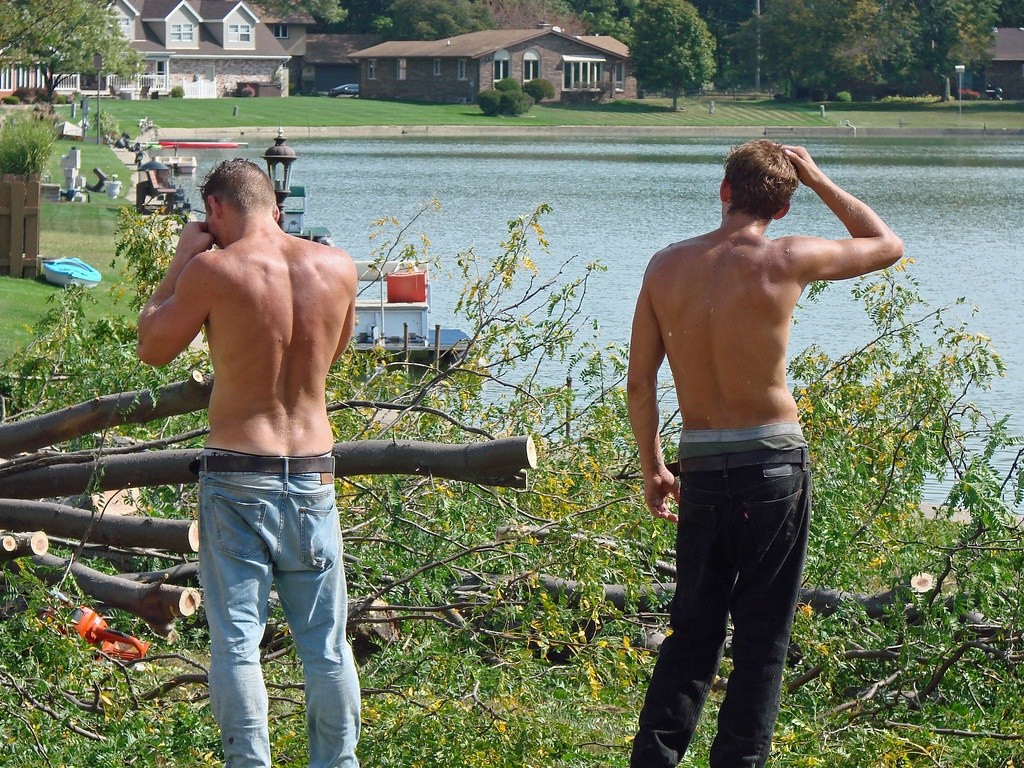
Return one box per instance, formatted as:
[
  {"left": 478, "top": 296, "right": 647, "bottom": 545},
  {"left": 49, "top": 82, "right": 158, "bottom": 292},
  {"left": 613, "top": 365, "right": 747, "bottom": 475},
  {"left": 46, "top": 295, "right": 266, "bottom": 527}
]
[{"left": 145, "top": 170, "right": 177, "bottom": 210}]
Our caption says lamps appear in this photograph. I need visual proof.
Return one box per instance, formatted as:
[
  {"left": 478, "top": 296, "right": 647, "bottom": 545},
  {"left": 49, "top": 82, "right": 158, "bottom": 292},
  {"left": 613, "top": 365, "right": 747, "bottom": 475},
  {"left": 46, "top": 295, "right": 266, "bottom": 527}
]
[{"left": 260, "top": 126, "right": 298, "bottom": 210}]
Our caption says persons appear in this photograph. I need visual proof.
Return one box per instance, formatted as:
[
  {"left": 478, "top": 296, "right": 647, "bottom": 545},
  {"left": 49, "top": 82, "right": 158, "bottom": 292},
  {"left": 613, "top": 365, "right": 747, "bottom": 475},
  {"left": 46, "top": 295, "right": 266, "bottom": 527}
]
[
  {"left": 627, "top": 138, "right": 904, "bottom": 768},
  {"left": 137, "top": 159, "right": 361, "bottom": 768}
]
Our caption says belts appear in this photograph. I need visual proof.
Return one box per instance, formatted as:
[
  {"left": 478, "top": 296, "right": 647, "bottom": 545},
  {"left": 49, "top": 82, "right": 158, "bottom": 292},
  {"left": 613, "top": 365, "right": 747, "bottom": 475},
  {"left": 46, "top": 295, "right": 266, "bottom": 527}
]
[
  {"left": 197, "top": 455, "right": 335, "bottom": 474},
  {"left": 678, "top": 448, "right": 803, "bottom": 473}
]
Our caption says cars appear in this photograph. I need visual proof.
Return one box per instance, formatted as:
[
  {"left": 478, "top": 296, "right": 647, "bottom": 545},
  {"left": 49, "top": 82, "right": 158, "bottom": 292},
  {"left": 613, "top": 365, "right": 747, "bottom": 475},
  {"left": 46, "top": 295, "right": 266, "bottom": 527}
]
[{"left": 330, "top": 83, "right": 360, "bottom": 102}]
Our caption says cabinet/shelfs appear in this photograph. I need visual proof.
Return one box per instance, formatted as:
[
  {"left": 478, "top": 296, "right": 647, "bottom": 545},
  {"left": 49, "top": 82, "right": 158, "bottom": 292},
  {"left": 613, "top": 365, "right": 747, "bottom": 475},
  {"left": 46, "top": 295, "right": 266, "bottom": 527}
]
[{"left": 354, "top": 299, "right": 429, "bottom": 342}]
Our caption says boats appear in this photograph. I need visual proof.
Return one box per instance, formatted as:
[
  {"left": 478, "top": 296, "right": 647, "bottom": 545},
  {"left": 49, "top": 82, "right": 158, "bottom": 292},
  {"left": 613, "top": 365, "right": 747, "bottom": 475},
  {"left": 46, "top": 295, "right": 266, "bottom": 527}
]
[{"left": 155, "top": 142, "right": 196, "bottom": 173}]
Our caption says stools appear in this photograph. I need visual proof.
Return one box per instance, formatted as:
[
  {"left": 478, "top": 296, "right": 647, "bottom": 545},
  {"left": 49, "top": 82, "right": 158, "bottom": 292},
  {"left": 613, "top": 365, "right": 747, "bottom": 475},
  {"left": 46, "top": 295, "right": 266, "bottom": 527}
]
[{"left": 86, "top": 167, "right": 106, "bottom": 193}]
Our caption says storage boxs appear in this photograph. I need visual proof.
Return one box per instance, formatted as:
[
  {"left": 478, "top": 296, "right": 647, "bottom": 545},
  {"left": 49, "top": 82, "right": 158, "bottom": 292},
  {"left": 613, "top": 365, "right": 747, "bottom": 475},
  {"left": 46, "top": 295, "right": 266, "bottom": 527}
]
[
  {"left": 39, "top": 149, "right": 86, "bottom": 203},
  {"left": 385, "top": 272, "right": 427, "bottom": 303}
]
